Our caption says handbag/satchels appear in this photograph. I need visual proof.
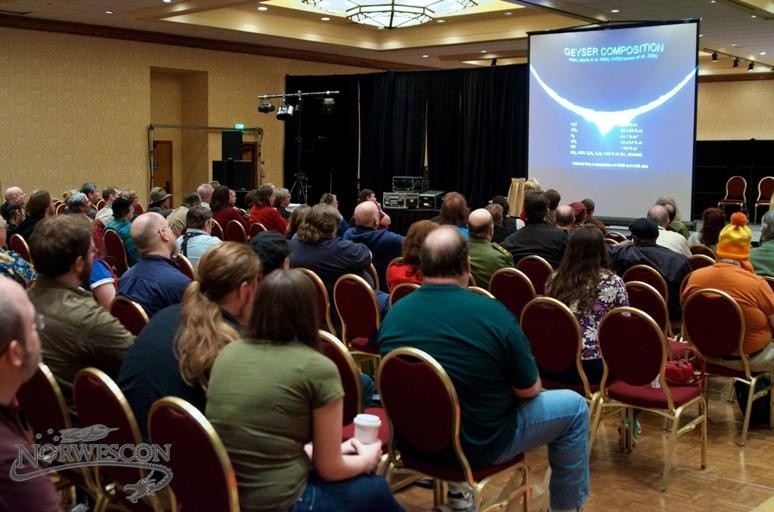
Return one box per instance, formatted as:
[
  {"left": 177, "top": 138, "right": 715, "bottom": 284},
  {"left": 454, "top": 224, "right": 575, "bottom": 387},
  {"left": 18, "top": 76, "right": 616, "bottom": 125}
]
[{"left": 666, "top": 347, "right": 705, "bottom": 385}]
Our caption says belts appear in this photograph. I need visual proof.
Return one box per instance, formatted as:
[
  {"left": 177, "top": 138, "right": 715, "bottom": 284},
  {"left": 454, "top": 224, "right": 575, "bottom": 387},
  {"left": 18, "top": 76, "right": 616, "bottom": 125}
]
[{"left": 722, "top": 343, "right": 768, "bottom": 360}]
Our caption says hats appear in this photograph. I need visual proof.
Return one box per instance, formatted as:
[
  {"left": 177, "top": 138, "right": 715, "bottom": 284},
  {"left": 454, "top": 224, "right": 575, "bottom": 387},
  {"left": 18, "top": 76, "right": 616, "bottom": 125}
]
[
  {"left": 249, "top": 231, "right": 294, "bottom": 276},
  {"left": 716, "top": 213, "right": 752, "bottom": 259},
  {"left": 209, "top": 181, "right": 219, "bottom": 188},
  {"left": 149, "top": 186, "right": 172, "bottom": 205}
]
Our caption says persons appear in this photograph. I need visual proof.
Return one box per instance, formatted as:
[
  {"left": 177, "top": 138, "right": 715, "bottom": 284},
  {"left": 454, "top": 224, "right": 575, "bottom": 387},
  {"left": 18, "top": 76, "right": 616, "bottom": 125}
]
[
  {"left": 102, "top": 197, "right": 141, "bottom": 267},
  {"left": 544, "top": 228, "right": 631, "bottom": 386},
  {"left": 377, "top": 228, "right": 590, "bottom": 511},
  {"left": 249, "top": 183, "right": 288, "bottom": 235},
  {"left": 545, "top": 189, "right": 561, "bottom": 217},
  {"left": 500, "top": 191, "right": 570, "bottom": 270},
  {"left": 145, "top": 187, "right": 172, "bottom": 219},
  {"left": 553, "top": 205, "right": 575, "bottom": 232},
  {"left": 290, "top": 204, "right": 373, "bottom": 293},
  {"left": 175, "top": 207, "right": 222, "bottom": 273},
  {"left": 0, "top": 277, "right": 60, "bottom": 512},
  {"left": 274, "top": 186, "right": 291, "bottom": 218},
  {"left": 94, "top": 186, "right": 121, "bottom": 225},
  {"left": 117, "top": 242, "right": 260, "bottom": 445},
  {"left": 206, "top": 269, "right": 406, "bottom": 511},
  {"left": 120, "top": 189, "right": 144, "bottom": 215},
  {"left": 746, "top": 213, "right": 774, "bottom": 277},
  {"left": 5, "top": 186, "right": 26, "bottom": 205},
  {"left": 581, "top": 199, "right": 607, "bottom": 234},
  {"left": 25, "top": 215, "right": 135, "bottom": 405},
  {"left": 465, "top": 208, "right": 515, "bottom": 290},
  {"left": 80, "top": 182, "right": 99, "bottom": 219},
  {"left": 655, "top": 195, "right": 691, "bottom": 240},
  {"left": 209, "top": 185, "right": 247, "bottom": 233},
  {"left": 249, "top": 231, "right": 289, "bottom": 281},
  {"left": 320, "top": 193, "right": 338, "bottom": 210},
  {"left": 687, "top": 208, "right": 726, "bottom": 253},
  {"left": 166, "top": 192, "right": 201, "bottom": 235},
  {"left": 343, "top": 200, "right": 406, "bottom": 275},
  {"left": 438, "top": 192, "right": 469, "bottom": 239},
  {"left": 387, "top": 220, "right": 440, "bottom": 292},
  {"left": 493, "top": 196, "right": 526, "bottom": 231},
  {"left": 26, "top": 189, "right": 56, "bottom": 231},
  {"left": 645, "top": 205, "right": 692, "bottom": 259},
  {"left": 115, "top": 212, "right": 193, "bottom": 322},
  {"left": 678, "top": 213, "right": 774, "bottom": 373},
  {"left": 5, "top": 204, "right": 25, "bottom": 249},
  {"left": 487, "top": 204, "right": 512, "bottom": 243},
  {"left": 356, "top": 190, "right": 391, "bottom": 226},
  {"left": 615, "top": 217, "right": 692, "bottom": 326},
  {"left": 67, "top": 193, "right": 93, "bottom": 222},
  {"left": 197, "top": 181, "right": 236, "bottom": 209},
  {"left": 568, "top": 201, "right": 586, "bottom": 227}
]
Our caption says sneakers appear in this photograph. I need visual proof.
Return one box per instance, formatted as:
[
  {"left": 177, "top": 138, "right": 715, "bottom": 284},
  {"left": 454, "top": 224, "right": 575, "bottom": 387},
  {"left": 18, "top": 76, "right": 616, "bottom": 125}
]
[
  {"left": 618, "top": 416, "right": 637, "bottom": 447},
  {"left": 445, "top": 488, "right": 474, "bottom": 510}
]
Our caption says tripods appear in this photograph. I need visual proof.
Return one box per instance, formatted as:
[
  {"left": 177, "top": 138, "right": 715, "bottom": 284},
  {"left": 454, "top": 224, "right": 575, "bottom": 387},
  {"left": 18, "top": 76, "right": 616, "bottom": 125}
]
[{"left": 290, "top": 180, "right": 307, "bottom": 203}]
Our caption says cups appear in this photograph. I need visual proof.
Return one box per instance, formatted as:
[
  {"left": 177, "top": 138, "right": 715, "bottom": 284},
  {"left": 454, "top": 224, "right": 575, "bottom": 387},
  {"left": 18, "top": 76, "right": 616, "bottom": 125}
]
[{"left": 353, "top": 415, "right": 380, "bottom": 445}]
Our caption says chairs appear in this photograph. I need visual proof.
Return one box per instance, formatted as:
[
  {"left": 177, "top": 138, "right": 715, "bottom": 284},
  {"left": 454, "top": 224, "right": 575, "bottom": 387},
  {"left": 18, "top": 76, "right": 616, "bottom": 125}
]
[
  {"left": 295, "top": 264, "right": 335, "bottom": 335},
  {"left": 173, "top": 254, "right": 195, "bottom": 281},
  {"left": 623, "top": 264, "right": 682, "bottom": 338},
  {"left": 520, "top": 297, "right": 627, "bottom": 488},
  {"left": 763, "top": 275, "right": 774, "bottom": 291},
  {"left": 333, "top": 272, "right": 381, "bottom": 376},
  {"left": 754, "top": 176, "right": 774, "bottom": 223},
  {"left": 90, "top": 204, "right": 97, "bottom": 212},
  {"left": 9, "top": 234, "right": 33, "bottom": 263},
  {"left": 227, "top": 220, "right": 247, "bottom": 245},
  {"left": 717, "top": 175, "right": 747, "bottom": 213},
  {"left": 687, "top": 254, "right": 715, "bottom": 270},
  {"left": 587, "top": 306, "right": 707, "bottom": 493},
  {"left": 110, "top": 295, "right": 149, "bottom": 336},
  {"left": 53, "top": 199, "right": 65, "bottom": 217},
  {"left": 604, "top": 231, "right": 628, "bottom": 241},
  {"left": 682, "top": 288, "right": 773, "bottom": 446},
  {"left": 103, "top": 228, "right": 131, "bottom": 271},
  {"left": 17, "top": 363, "right": 99, "bottom": 512},
  {"left": 489, "top": 267, "right": 535, "bottom": 321},
  {"left": 603, "top": 238, "right": 618, "bottom": 246},
  {"left": 626, "top": 282, "right": 717, "bottom": 417},
  {"left": 135, "top": 202, "right": 143, "bottom": 215},
  {"left": 366, "top": 260, "right": 379, "bottom": 290},
  {"left": 468, "top": 286, "right": 496, "bottom": 300},
  {"left": 149, "top": 393, "right": 239, "bottom": 511},
  {"left": 388, "top": 282, "right": 421, "bottom": 310},
  {"left": 379, "top": 345, "right": 531, "bottom": 512},
  {"left": 251, "top": 222, "right": 267, "bottom": 239},
  {"left": 468, "top": 273, "right": 476, "bottom": 286},
  {"left": 313, "top": 328, "right": 424, "bottom": 492},
  {"left": 210, "top": 217, "right": 224, "bottom": 242},
  {"left": 77, "top": 367, "right": 164, "bottom": 512},
  {"left": 691, "top": 244, "right": 718, "bottom": 260},
  {"left": 516, "top": 254, "right": 554, "bottom": 298},
  {"left": 98, "top": 199, "right": 105, "bottom": 209}
]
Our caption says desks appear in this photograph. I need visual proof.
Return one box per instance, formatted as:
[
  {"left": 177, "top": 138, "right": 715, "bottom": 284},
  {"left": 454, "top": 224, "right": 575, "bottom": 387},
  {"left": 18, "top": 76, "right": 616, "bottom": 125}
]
[{"left": 384, "top": 209, "right": 442, "bottom": 236}]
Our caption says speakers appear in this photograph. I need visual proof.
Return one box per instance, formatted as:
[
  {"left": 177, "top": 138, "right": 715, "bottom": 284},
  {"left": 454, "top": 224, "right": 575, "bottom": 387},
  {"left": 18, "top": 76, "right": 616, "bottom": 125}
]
[
  {"left": 222, "top": 131, "right": 242, "bottom": 160},
  {"left": 233, "top": 160, "right": 254, "bottom": 190},
  {"left": 212, "top": 160, "right": 227, "bottom": 185}
]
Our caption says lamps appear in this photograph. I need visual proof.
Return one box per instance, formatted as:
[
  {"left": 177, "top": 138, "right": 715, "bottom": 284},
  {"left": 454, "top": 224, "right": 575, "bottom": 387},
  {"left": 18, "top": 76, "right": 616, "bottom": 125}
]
[
  {"left": 748, "top": 61, "right": 754, "bottom": 72},
  {"left": 733, "top": 57, "right": 738, "bottom": 68},
  {"left": 491, "top": 59, "right": 496, "bottom": 65},
  {"left": 303, "top": 0, "right": 494, "bottom": 30},
  {"left": 712, "top": 53, "right": 718, "bottom": 62}
]
